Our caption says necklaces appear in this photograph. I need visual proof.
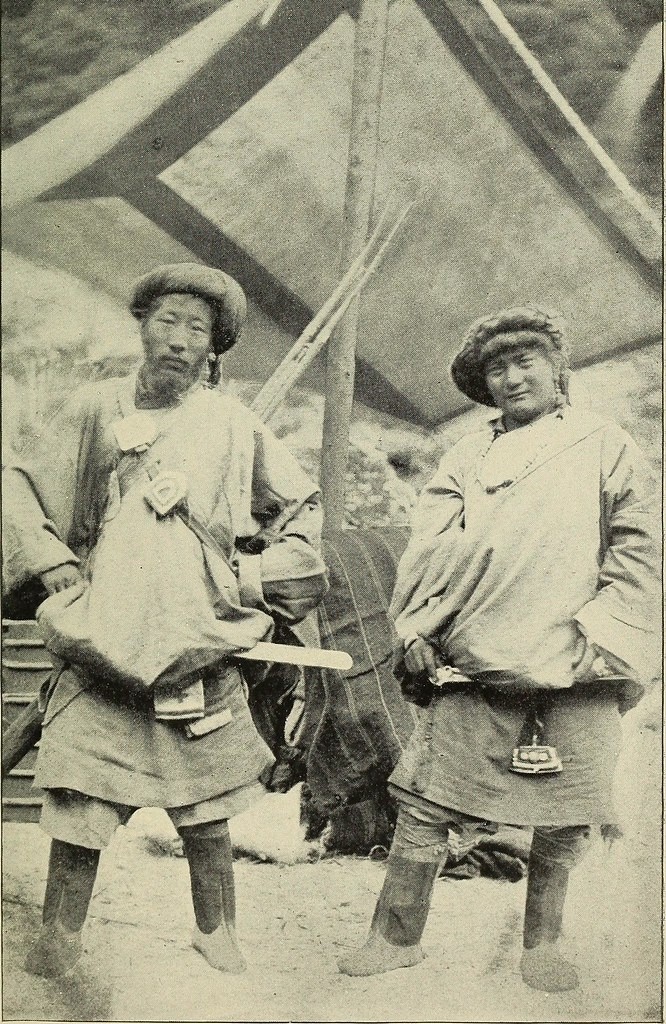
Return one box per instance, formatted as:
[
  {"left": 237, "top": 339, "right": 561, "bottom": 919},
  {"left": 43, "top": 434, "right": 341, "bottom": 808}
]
[{"left": 474, "top": 402, "right": 569, "bottom": 494}]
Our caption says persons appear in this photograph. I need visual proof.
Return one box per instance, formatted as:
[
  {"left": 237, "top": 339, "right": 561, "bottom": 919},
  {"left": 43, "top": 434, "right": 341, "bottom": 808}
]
[
  {"left": 334, "top": 308, "right": 664, "bottom": 992},
  {"left": 1, "top": 263, "right": 328, "bottom": 981}
]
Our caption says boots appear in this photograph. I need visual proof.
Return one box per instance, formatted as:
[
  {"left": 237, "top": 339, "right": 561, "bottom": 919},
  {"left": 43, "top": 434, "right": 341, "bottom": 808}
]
[
  {"left": 521, "top": 854, "right": 577, "bottom": 990},
  {"left": 182, "top": 833, "right": 247, "bottom": 973},
  {"left": 25, "top": 840, "right": 101, "bottom": 977},
  {"left": 337, "top": 856, "right": 441, "bottom": 977}
]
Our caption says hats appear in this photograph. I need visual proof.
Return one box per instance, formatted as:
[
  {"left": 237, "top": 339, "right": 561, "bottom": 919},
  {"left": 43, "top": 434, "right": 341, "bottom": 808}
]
[
  {"left": 451, "top": 308, "right": 570, "bottom": 407},
  {"left": 128, "top": 264, "right": 247, "bottom": 356}
]
